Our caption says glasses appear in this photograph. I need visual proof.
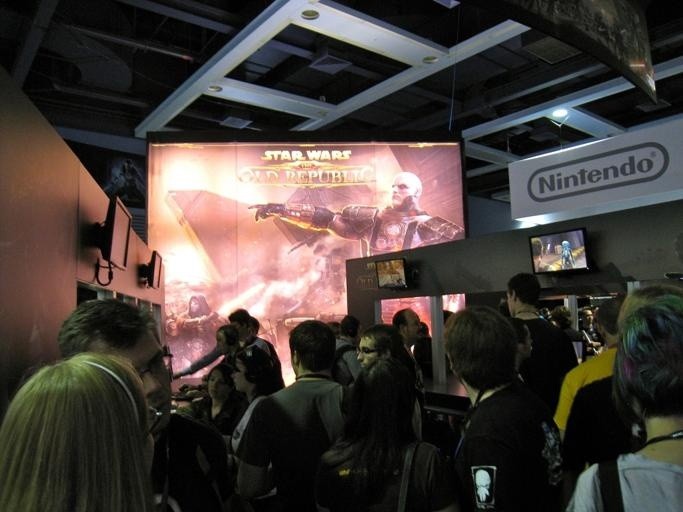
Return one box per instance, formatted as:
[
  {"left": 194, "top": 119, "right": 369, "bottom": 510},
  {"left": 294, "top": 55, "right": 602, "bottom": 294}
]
[
  {"left": 357, "top": 347, "right": 378, "bottom": 354},
  {"left": 141, "top": 346, "right": 173, "bottom": 438}
]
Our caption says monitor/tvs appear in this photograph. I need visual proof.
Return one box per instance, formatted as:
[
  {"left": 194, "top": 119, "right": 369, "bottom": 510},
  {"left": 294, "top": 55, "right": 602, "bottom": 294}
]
[
  {"left": 147, "top": 250, "right": 163, "bottom": 289},
  {"left": 528, "top": 228, "right": 590, "bottom": 275},
  {"left": 101, "top": 196, "right": 133, "bottom": 271},
  {"left": 375, "top": 258, "right": 409, "bottom": 289}
]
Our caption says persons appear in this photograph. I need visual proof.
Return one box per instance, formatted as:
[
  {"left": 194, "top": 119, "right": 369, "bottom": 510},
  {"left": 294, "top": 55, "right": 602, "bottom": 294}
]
[
  {"left": 500, "top": 274, "right": 682, "bottom": 440},
  {"left": 248, "top": 171, "right": 465, "bottom": 258},
  {"left": 57, "top": 299, "right": 237, "bottom": 510},
  {"left": 568, "top": 306, "right": 683, "bottom": 510},
  {"left": 2, "top": 354, "right": 162, "bottom": 510},
  {"left": 560, "top": 240, "right": 576, "bottom": 270},
  {"left": 531, "top": 238, "right": 555, "bottom": 272},
  {"left": 445, "top": 306, "right": 564, "bottom": 511},
  {"left": 177, "top": 310, "right": 433, "bottom": 512}
]
